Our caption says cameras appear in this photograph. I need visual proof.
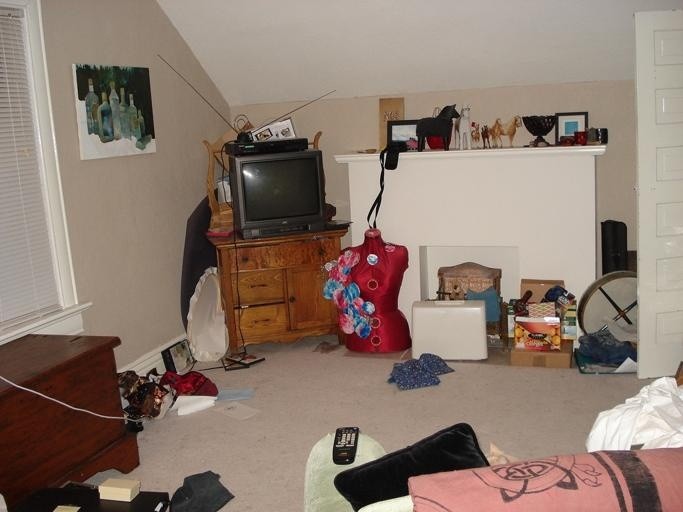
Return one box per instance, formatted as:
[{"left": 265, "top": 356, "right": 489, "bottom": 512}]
[{"left": 384, "top": 141, "right": 407, "bottom": 170}]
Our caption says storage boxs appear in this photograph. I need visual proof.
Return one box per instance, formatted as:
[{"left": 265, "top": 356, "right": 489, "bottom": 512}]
[
  {"left": 514, "top": 279, "right": 564, "bottom": 352},
  {"left": 15, "top": 488, "right": 169, "bottom": 512},
  {"left": 507, "top": 299, "right": 577, "bottom": 339},
  {"left": 412, "top": 300, "right": 488, "bottom": 360},
  {"left": 511, "top": 340, "right": 573, "bottom": 368}
]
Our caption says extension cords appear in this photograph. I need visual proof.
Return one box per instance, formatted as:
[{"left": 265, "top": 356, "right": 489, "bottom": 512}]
[{"left": 127, "top": 422, "right": 143, "bottom": 432}]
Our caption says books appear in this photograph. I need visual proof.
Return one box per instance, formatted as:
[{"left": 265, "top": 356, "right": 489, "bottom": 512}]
[{"left": 560, "top": 300, "right": 577, "bottom": 340}]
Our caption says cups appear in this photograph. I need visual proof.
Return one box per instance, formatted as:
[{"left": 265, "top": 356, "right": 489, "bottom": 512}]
[{"left": 559, "top": 127, "right": 608, "bottom": 147}]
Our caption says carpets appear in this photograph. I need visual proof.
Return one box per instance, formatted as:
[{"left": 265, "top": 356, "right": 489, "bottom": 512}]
[{"left": 575, "top": 341, "right": 636, "bottom": 374}]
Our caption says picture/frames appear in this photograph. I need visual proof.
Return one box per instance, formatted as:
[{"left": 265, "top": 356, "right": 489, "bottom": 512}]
[
  {"left": 387, "top": 120, "right": 425, "bottom": 149},
  {"left": 221, "top": 358, "right": 249, "bottom": 371},
  {"left": 226, "top": 352, "right": 265, "bottom": 366},
  {"left": 555, "top": 112, "right": 588, "bottom": 146}
]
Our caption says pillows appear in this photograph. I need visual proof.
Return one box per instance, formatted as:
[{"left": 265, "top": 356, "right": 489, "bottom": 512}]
[{"left": 334, "top": 423, "right": 523, "bottom": 511}]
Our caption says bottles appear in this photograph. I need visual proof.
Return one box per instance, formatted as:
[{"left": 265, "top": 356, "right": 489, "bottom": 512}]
[
  {"left": 515, "top": 290, "right": 532, "bottom": 314},
  {"left": 506, "top": 299, "right": 514, "bottom": 315},
  {"left": 84, "top": 78, "right": 152, "bottom": 150}
]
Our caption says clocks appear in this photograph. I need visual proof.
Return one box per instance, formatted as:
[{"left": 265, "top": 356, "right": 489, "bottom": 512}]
[{"left": 578, "top": 271, "right": 637, "bottom": 342}]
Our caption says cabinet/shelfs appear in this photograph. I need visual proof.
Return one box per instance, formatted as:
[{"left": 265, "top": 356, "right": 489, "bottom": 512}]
[
  {"left": 207, "top": 229, "right": 348, "bottom": 357},
  {"left": 0, "top": 334, "right": 139, "bottom": 512}
]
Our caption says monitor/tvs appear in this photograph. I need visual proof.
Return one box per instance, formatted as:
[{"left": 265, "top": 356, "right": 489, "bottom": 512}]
[{"left": 227, "top": 148, "right": 327, "bottom": 239}]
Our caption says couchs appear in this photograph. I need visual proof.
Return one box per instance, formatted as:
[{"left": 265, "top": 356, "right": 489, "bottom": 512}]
[{"left": 304, "top": 434, "right": 682, "bottom": 511}]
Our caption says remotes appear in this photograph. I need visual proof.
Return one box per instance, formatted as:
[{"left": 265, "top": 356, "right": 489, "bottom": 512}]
[{"left": 333, "top": 426, "right": 358, "bottom": 465}]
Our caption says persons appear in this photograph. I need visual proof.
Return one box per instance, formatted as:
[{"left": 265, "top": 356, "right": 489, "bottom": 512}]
[{"left": 320, "top": 228, "right": 411, "bottom": 354}]
